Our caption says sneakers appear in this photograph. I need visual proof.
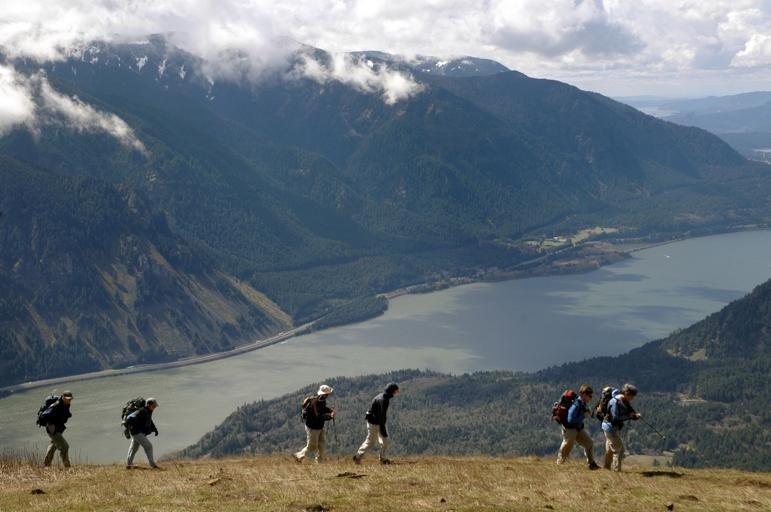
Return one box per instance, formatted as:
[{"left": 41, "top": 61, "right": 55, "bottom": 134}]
[
  {"left": 589, "top": 461, "right": 601, "bottom": 470},
  {"left": 126, "top": 464, "right": 162, "bottom": 470},
  {"left": 292, "top": 452, "right": 303, "bottom": 465},
  {"left": 353, "top": 456, "right": 361, "bottom": 466},
  {"left": 381, "top": 459, "right": 395, "bottom": 465}
]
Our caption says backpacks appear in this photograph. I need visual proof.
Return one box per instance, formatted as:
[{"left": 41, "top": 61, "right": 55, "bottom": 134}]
[
  {"left": 121, "top": 396, "right": 145, "bottom": 428},
  {"left": 36, "top": 395, "right": 59, "bottom": 426},
  {"left": 301, "top": 395, "right": 317, "bottom": 420},
  {"left": 551, "top": 390, "right": 577, "bottom": 424},
  {"left": 596, "top": 386, "right": 615, "bottom": 422}
]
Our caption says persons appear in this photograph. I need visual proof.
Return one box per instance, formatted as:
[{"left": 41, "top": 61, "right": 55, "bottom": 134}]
[
  {"left": 38, "top": 391, "right": 73, "bottom": 466},
  {"left": 125, "top": 397, "right": 162, "bottom": 470},
  {"left": 294, "top": 384, "right": 336, "bottom": 463},
  {"left": 601, "top": 383, "right": 643, "bottom": 472},
  {"left": 556, "top": 384, "right": 603, "bottom": 470},
  {"left": 353, "top": 383, "right": 400, "bottom": 464}
]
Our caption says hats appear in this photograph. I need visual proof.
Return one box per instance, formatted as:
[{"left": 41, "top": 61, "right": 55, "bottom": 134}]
[
  {"left": 146, "top": 398, "right": 160, "bottom": 406},
  {"left": 317, "top": 385, "right": 333, "bottom": 395},
  {"left": 62, "top": 391, "right": 73, "bottom": 400}
]
